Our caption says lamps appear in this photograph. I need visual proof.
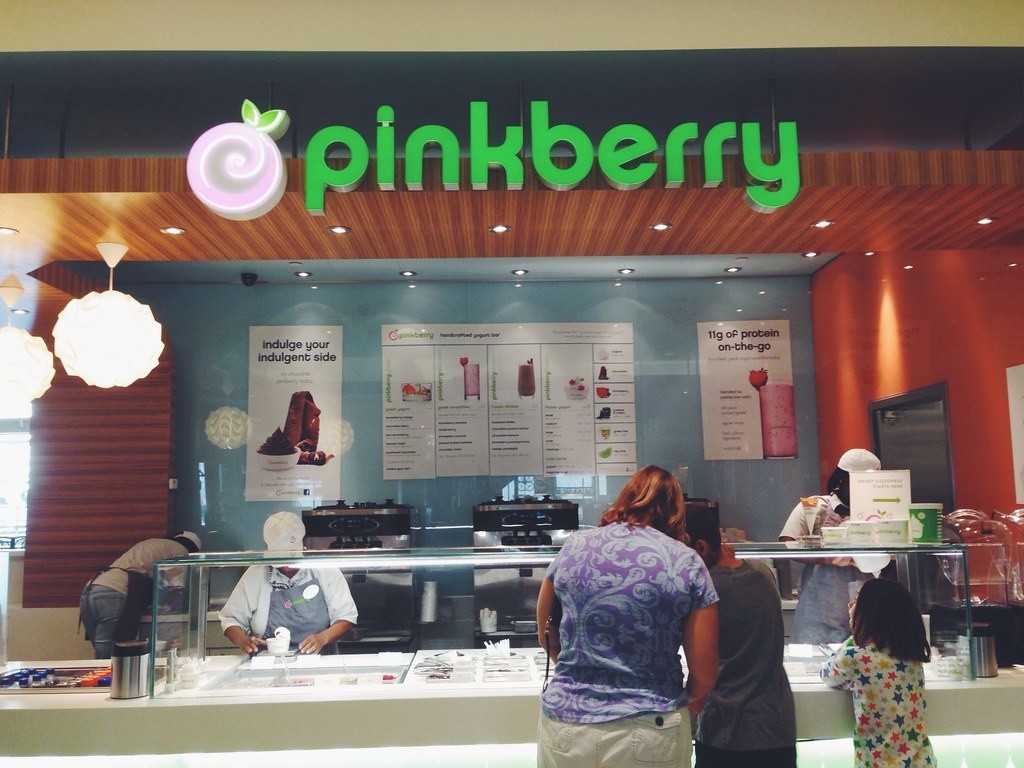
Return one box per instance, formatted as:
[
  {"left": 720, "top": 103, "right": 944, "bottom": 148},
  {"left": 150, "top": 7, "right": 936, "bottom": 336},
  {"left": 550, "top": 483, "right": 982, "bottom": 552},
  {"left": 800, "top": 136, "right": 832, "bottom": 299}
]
[
  {"left": 51, "top": 242, "right": 165, "bottom": 388},
  {"left": 0, "top": 243, "right": 56, "bottom": 409}
]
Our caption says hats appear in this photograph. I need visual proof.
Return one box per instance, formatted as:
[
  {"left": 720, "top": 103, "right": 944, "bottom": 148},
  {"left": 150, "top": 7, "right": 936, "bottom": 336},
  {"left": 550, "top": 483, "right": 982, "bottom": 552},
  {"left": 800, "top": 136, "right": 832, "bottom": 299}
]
[
  {"left": 263, "top": 511, "right": 306, "bottom": 568},
  {"left": 174, "top": 530, "right": 201, "bottom": 550},
  {"left": 837, "top": 448, "right": 882, "bottom": 472}
]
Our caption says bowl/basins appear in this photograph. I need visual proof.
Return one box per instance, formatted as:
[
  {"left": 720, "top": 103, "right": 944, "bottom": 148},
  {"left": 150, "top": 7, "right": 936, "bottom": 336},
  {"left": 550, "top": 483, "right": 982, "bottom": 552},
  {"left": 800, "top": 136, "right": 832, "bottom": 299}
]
[
  {"left": 874, "top": 518, "right": 911, "bottom": 547},
  {"left": 821, "top": 526, "right": 848, "bottom": 545},
  {"left": 266, "top": 637, "right": 291, "bottom": 655},
  {"left": 253, "top": 446, "right": 300, "bottom": 471},
  {"left": 845, "top": 520, "right": 876, "bottom": 548},
  {"left": 564, "top": 385, "right": 589, "bottom": 400},
  {"left": 400, "top": 382, "right": 433, "bottom": 402}
]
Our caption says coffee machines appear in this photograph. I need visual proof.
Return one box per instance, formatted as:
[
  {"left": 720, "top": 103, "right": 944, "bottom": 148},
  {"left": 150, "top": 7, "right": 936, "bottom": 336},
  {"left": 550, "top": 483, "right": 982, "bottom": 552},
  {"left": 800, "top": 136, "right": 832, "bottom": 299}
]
[
  {"left": 296, "top": 497, "right": 418, "bottom": 655},
  {"left": 472, "top": 493, "right": 580, "bottom": 649}
]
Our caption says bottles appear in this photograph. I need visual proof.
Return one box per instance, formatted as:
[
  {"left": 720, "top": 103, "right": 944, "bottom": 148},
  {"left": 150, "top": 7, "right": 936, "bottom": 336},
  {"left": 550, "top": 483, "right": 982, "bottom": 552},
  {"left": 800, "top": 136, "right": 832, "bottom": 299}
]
[{"left": 1, "top": 665, "right": 113, "bottom": 688}]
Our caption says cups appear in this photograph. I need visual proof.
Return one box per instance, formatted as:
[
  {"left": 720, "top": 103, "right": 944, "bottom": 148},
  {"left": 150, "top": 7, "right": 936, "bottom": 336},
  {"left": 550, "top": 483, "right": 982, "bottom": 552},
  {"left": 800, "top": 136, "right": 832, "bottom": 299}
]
[
  {"left": 419, "top": 581, "right": 437, "bottom": 622},
  {"left": 759, "top": 385, "right": 797, "bottom": 460},
  {"left": 463, "top": 364, "right": 480, "bottom": 401},
  {"left": 480, "top": 609, "right": 498, "bottom": 634},
  {"left": 517, "top": 364, "right": 535, "bottom": 399}
]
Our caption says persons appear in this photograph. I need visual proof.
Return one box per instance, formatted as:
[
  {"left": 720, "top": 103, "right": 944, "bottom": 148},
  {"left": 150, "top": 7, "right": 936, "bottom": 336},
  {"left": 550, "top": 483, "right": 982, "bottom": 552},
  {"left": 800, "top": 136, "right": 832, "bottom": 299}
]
[
  {"left": 536, "top": 463, "right": 719, "bottom": 768},
  {"left": 820, "top": 578, "right": 937, "bottom": 768},
  {"left": 778, "top": 448, "right": 899, "bottom": 644},
  {"left": 684, "top": 508, "right": 797, "bottom": 768},
  {"left": 77, "top": 530, "right": 202, "bottom": 659},
  {"left": 218, "top": 511, "right": 358, "bottom": 660}
]
